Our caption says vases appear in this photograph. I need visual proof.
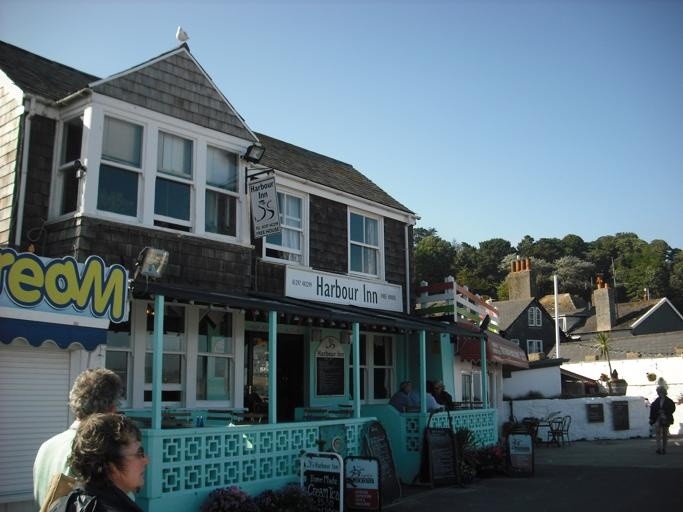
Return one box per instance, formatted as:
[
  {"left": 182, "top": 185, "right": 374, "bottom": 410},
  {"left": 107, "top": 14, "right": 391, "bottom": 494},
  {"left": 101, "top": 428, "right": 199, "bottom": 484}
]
[{"left": 476, "top": 467, "right": 504, "bottom": 478}]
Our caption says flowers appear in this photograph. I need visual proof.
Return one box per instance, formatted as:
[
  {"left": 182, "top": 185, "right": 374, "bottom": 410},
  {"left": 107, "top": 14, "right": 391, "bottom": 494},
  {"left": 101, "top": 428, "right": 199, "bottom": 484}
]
[{"left": 468, "top": 444, "right": 507, "bottom": 467}]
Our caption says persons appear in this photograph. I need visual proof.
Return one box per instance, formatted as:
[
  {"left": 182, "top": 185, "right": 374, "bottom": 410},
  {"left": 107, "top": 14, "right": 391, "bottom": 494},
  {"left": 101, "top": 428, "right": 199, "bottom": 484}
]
[
  {"left": 389, "top": 379, "right": 453, "bottom": 412},
  {"left": 649, "top": 386, "right": 675, "bottom": 454},
  {"left": 32, "top": 368, "right": 136, "bottom": 512},
  {"left": 47, "top": 412, "right": 149, "bottom": 512}
]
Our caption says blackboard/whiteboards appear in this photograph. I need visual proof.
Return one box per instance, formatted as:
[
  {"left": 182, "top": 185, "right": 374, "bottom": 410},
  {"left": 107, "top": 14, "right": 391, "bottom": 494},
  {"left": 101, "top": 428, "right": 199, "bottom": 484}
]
[
  {"left": 364, "top": 420, "right": 401, "bottom": 506},
  {"left": 317, "top": 357, "right": 344, "bottom": 395},
  {"left": 427, "top": 428, "right": 459, "bottom": 481}
]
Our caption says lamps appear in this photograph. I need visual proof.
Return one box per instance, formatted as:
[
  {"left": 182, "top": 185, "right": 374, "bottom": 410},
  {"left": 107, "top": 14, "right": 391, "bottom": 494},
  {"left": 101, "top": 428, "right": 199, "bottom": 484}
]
[
  {"left": 243, "top": 144, "right": 265, "bottom": 164},
  {"left": 138, "top": 246, "right": 169, "bottom": 279}
]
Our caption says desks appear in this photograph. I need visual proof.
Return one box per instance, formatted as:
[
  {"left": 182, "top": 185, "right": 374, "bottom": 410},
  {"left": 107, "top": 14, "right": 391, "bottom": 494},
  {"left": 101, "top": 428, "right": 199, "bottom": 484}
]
[
  {"left": 303, "top": 405, "right": 355, "bottom": 419},
  {"left": 130, "top": 407, "right": 271, "bottom": 429}
]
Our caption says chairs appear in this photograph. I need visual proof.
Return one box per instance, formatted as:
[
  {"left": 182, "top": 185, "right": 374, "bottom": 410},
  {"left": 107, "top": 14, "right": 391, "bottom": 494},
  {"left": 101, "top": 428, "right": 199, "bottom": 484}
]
[{"left": 546, "top": 415, "right": 573, "bottom": 450}]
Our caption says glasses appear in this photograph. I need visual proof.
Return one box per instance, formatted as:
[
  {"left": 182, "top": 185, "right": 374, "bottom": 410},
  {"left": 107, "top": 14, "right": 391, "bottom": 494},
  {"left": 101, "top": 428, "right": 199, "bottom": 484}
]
[{"left": 120, "top": 445, "right": 146, "bottom": 460}]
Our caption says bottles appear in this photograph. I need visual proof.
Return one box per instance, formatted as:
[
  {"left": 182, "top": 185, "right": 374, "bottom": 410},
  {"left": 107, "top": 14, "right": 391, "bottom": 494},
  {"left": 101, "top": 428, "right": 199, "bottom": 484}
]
[
  {"left": 236, "top": 416, "right": 264, "bottom": 423},
  {"left": 195, "top": 415, "right": 203, "bottom": 427}
]
